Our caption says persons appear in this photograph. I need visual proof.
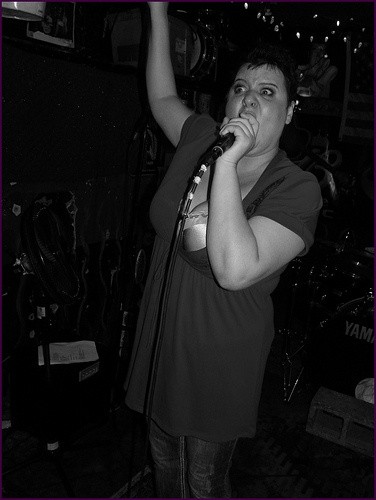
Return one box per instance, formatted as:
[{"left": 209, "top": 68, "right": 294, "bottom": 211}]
[{"left": 122, "top": 1, "right": 323, "bottom": 500}]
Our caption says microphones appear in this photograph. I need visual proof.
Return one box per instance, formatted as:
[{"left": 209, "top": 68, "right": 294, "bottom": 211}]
[{"left": 199, "top": 133, "right": 235, "bottom": 173}]
